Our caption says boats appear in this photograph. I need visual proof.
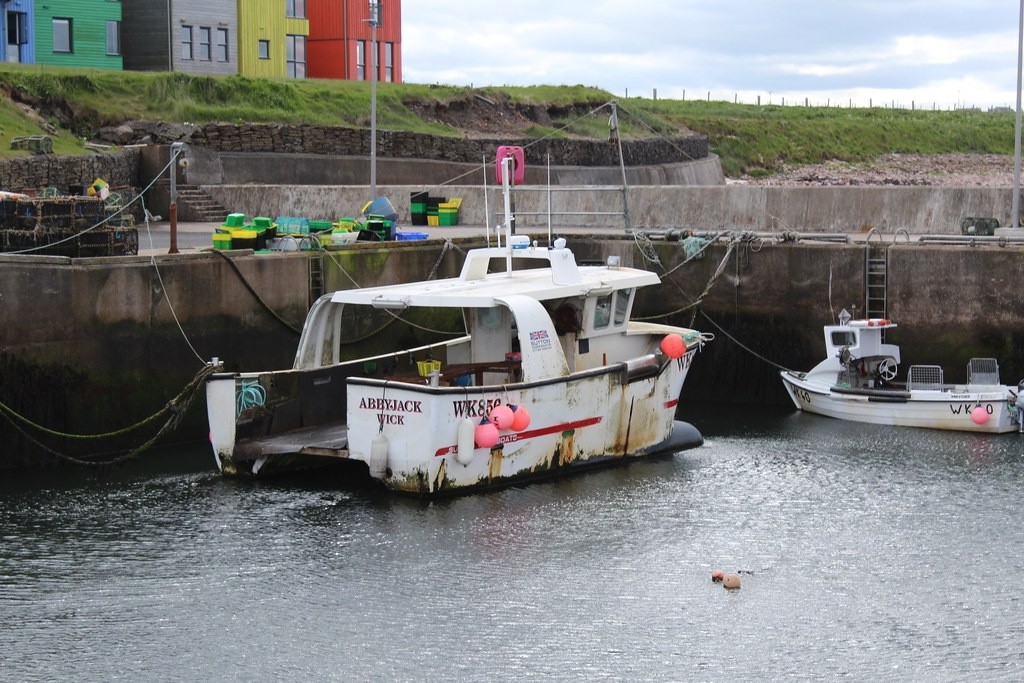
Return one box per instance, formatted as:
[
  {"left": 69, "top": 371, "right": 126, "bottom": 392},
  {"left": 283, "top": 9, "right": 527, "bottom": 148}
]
[
  {"left": 205, "top": 146, "right": 706, "bottom": 503},
  {"left": 781, "top": 305, "right": 1024, "bottom": 435}
]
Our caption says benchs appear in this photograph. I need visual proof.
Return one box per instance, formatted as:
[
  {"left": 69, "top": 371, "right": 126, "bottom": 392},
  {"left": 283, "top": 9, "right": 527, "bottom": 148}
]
[{"left": 385, "top": 361, "right": 521, "bottom": 386}]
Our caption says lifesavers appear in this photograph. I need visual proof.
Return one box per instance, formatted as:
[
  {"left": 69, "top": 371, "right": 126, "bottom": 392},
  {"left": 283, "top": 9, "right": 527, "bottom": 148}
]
[{"left": 869, "top": 317, "right": 891, "bottom": 328}]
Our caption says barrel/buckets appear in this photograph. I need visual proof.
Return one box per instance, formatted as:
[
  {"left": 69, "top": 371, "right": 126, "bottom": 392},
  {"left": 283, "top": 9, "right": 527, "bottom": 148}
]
[{"left": 363, "top": 196, "right": 398, "bottom": 240}]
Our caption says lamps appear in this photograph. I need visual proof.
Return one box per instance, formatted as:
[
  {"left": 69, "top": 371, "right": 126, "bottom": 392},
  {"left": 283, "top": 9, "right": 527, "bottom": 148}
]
[{"left": 607, "top": 256, "right": 621, "bottom": 270}]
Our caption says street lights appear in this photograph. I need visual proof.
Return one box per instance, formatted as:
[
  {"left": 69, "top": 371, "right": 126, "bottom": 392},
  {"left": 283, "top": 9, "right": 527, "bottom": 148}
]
[{"left": 362, "top": 19, "right": 376, "bottom": 197}]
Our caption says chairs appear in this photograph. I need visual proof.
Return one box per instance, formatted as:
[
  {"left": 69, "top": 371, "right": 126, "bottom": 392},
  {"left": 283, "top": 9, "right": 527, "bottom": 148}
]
[{"left": 258, "top": 373, "right": 303, "bottom": 435}]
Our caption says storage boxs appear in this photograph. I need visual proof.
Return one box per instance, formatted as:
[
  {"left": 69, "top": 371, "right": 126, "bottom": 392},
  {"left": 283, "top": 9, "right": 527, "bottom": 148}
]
[
  {"left": 411, "top": 192, "right": 463, "bottom": 226},
  {"left": 212, "top": 212, "right": 429, "bottom": 254}
]
